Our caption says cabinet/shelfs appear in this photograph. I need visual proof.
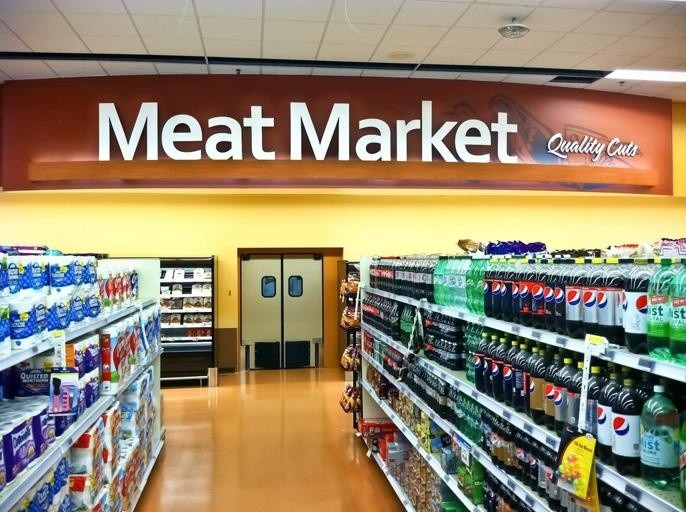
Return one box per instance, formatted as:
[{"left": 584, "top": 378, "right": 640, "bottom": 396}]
[
  {"left": 1, "top": 298, "right": 166, "bottom": 512},
  {"left": 358, "top": 256, "right": 685, "bottom": 512},
  {"left": 161, "top": 258, "right": 214, "bottom": 386}
]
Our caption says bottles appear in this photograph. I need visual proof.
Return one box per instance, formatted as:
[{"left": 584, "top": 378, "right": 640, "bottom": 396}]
[{"left": 362, "top": 255, "right": 685, "bottom": 511}]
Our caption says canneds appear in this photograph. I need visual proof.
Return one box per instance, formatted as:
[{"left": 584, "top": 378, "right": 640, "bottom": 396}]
[{"left": 364, "top": 331, "right": 388, "bottom": 366}]
